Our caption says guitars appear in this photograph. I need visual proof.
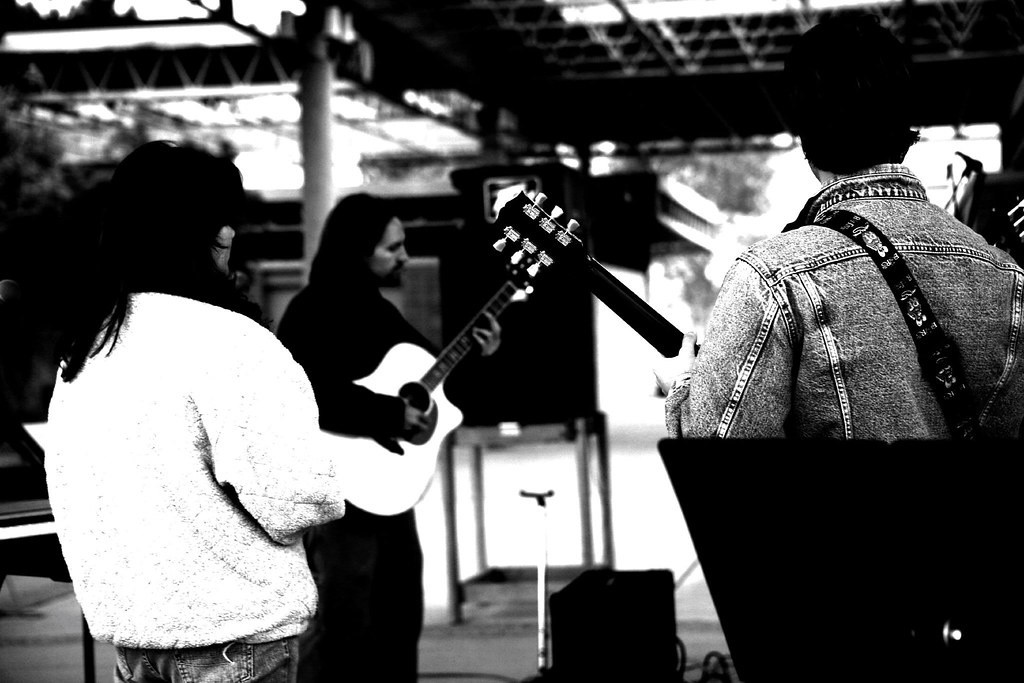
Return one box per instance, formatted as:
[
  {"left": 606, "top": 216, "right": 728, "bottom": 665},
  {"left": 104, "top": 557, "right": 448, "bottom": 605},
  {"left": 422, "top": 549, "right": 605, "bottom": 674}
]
[
  {"left": 317, "top": 277, "right": 528, "bottom": 517},
  {"left": 491, "top": 190, "right": 702, "bottom": 367}
]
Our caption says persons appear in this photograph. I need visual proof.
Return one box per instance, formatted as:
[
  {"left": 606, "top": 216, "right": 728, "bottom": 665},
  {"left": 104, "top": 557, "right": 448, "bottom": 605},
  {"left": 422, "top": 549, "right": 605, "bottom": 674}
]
[
  {"left": 654, "top": 17, "right": 1024, "bottom": 438},
  {"left": 278, "top": 191, "right": 502, "bottom": 683},
  {"left": 44, "top": 139, "right": 346, "bottom": 683}
]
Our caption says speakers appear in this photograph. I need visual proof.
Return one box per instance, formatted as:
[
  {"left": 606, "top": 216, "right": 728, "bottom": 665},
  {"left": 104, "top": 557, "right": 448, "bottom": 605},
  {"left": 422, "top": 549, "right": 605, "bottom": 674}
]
[
  {"left": 550, "top": 567, "right": 680, "bottom": 683},
  {"left": 434, "top": 249, "right": 598, "bottom": 427}
]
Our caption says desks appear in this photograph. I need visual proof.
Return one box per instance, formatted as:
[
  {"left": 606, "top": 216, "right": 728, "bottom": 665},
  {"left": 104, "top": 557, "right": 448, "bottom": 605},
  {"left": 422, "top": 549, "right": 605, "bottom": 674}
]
[{"left": 439, "top": 414, "right": 612, "bottom": 629}]
